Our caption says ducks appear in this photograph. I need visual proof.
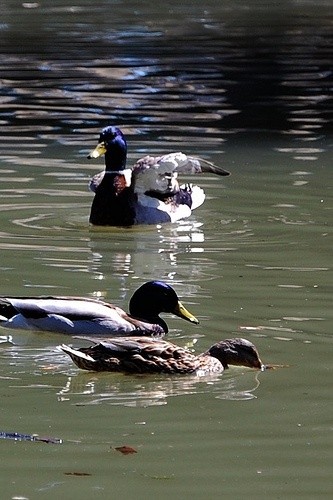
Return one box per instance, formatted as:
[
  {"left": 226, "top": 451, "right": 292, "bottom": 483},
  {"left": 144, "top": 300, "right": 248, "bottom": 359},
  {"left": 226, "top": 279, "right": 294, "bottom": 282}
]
[
  {"left": 0, "top": 278, "right": 200, "bottom": 338},
  {"left": 85, "top": 126, "right": 230, "bottom": 227},
  {"left": 55, "top": 336, "right": 271, "bottom": 379}
]
